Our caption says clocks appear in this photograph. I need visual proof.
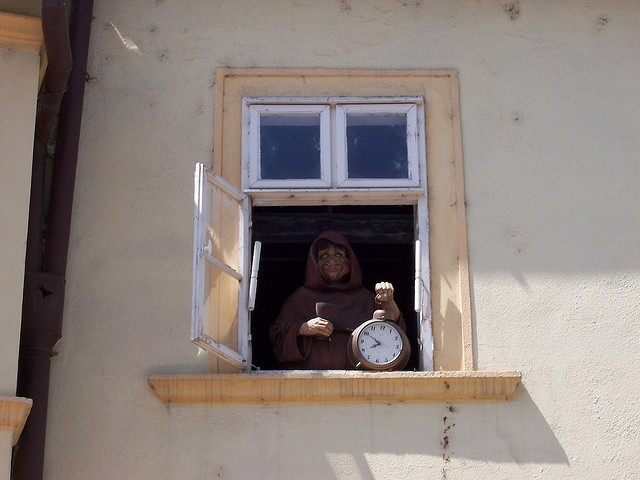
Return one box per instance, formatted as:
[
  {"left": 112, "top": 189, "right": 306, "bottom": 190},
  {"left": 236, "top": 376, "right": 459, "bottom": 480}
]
[{"left": 350, "top": 309, "right": 412, "bottom": 371}]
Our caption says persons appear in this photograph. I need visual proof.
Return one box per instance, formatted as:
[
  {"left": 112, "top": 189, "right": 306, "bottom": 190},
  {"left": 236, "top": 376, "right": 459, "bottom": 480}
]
[{"left": 269, "top": 230, "right": 407, "bottom": 370}]
